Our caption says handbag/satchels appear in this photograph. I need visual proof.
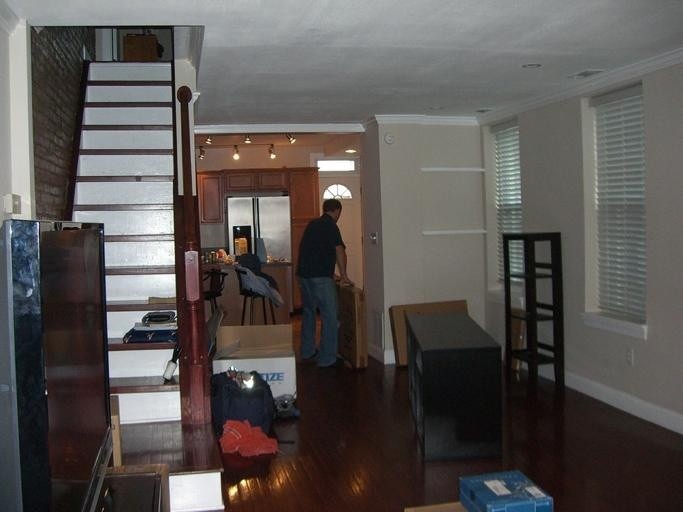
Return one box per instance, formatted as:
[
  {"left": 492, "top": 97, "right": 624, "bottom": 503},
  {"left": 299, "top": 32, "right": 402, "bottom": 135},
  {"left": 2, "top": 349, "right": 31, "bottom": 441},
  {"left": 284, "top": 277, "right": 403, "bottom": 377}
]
[
  {"left": 210, "top": 370, "right": 273, "bottom": 436},
  {"left": 143, "top": 311, "right": 175, "bottom": 324},
  {"left": 122, "top": 328, "right": 177, "bottom": 344}
]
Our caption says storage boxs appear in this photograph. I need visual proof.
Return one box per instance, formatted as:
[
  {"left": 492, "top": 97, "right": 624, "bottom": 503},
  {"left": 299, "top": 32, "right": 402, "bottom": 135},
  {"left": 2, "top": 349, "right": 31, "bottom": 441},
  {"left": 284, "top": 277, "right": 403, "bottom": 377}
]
[{"left": 212, "top": 324, "right": 298, "bottom": 398}]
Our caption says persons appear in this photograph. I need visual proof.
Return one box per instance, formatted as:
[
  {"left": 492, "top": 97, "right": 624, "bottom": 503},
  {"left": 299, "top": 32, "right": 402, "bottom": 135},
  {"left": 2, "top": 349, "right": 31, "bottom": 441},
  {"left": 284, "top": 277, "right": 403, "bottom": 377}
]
[{"left": 292, "top": 197, "right": 355, "bottom": 370}]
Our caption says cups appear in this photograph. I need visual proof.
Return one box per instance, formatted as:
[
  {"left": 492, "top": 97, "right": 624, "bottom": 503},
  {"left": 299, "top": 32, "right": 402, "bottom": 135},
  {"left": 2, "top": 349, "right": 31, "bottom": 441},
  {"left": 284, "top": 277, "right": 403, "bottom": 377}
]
[{"left": 266, "top": 256, "right": 272, "bottom": 265}]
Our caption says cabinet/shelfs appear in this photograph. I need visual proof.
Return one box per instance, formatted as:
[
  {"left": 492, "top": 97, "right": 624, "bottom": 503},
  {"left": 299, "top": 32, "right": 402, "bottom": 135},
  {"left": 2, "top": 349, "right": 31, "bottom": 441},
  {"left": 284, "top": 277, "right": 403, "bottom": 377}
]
[
  {"left": 123, "top": 35, "right": 158, "bottom": 60},
  {"left": 105, "top": 462, "right": 170, "bottom": 511},
  {"left": 502, "top": 231, "right": 565, "bottom": 385},
  {"left": 223, "top": 168, "right": 288, "bottom": 192},
  {"left": 197, "top": 171, "right": 224, "bottom": 225}
]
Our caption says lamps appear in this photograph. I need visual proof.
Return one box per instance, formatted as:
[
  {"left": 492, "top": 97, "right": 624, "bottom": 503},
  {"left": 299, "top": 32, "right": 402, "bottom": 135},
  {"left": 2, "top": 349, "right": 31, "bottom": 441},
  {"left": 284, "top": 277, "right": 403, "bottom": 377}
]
[{"left": 195, "top": 134, "right": 296, "bottom": 160}]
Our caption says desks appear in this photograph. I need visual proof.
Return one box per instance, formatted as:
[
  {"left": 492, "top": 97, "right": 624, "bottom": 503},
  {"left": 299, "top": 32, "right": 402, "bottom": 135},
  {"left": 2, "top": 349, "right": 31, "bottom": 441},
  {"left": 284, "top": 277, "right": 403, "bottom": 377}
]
[{"left": 404, "top": 310, "right": 501, "bottom": 463}]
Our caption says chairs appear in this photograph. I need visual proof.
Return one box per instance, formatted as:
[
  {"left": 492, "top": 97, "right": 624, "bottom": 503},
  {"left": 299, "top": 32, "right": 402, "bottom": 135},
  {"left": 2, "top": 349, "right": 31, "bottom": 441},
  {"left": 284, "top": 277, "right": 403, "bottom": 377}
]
[
  {"left": 233, "top": 254, "right": 283, "bottom": 325},
  {"left": 203, "top": 271, "right": 228, "bottom": 313}
]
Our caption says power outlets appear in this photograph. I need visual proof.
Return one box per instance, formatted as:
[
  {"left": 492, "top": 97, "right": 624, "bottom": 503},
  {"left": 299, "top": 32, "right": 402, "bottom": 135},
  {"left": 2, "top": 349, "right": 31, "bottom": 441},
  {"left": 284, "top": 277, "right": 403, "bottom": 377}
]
[{"left": 625, "top": 348, "right": 633, "bottom": 366}]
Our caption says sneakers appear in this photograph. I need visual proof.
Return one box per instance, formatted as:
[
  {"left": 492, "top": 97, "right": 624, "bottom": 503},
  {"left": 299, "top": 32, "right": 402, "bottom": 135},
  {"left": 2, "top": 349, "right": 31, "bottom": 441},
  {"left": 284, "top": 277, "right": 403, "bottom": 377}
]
[{"left": 303, "top": 349, "right": 344, "bottom": 369}]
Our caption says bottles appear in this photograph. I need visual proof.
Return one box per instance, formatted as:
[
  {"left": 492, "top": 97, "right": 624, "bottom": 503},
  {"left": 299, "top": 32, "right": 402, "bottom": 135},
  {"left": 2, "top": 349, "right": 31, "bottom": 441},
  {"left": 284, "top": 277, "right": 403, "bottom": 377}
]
[{"left": 204, "top": 249, "right": 224, "bottom": 265}]
